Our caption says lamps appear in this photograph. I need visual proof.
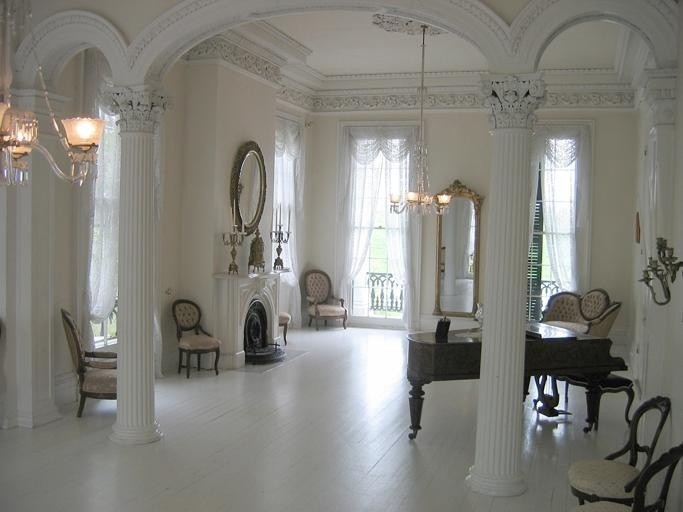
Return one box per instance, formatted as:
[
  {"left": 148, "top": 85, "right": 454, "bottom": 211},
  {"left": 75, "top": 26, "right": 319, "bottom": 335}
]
[
  {"left": 0, "top": 1, "right": 108, "bottom": 189},
  {"left": 387, "top": 24, "right": 453, "bottom": 217}
]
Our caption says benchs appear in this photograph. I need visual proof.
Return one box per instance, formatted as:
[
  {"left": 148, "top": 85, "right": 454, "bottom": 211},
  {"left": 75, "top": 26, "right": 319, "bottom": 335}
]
[{"left": 538, "top": 372, "right": 633, "bottom": 433}]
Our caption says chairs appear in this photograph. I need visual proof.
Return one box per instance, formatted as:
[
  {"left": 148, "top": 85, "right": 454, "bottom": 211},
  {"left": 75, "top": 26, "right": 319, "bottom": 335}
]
[
  {"left": 59, "top": 307, "right": 117, "bottom": 417},
  {"left": 567, "top": 393, "right": 672, "bottom": 505},
  {"left": 278, "top": 311, "right": 291, "bottom": 346},
  {"left": 303, "top": 269, "right": 347, "bottom": 332},
  {"left": 171, "top": 299, "right": 221, "bottom": 379},
  {"left": 567, "top": 442, "right": 683, "bottom": 511}
]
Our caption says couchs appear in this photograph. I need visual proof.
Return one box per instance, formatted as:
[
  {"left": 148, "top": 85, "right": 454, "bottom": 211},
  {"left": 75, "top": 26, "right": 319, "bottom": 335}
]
[{"left": 539, "top": 286, "right": 622, "bottom": 338}]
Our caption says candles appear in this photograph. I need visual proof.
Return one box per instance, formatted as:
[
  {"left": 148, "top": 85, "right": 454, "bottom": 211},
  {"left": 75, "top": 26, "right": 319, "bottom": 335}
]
[
  {"left": 278, "top": 203, "right": 281, "bottom": 225},
  {"left": 643, "top": 233, "right": 649, "bottom": 267},
  {"left": 241, "top": 207, "right": 244, "bottom": 233},
  {"left": 232, "top": 198, "right": 235, "bottom": 225},
  {"left": 275, "top": 208, "right": 277, "bottom": 231},
  {"left": 287, "top": 204, "right": 290, "bottom": 233},
  {"left": 229, "top": 207, "right": 232, "bottom": 234},
  {"left": 270, "top": 210, "right": 273, "bottom": 232}
]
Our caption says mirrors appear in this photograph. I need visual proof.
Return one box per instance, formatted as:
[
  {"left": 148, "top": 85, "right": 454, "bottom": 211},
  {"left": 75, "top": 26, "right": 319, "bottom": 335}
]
[
  {"left": 432, "top": 179, "right": 486, "bottom": 318},
  {"left": 229, "top": 140, "right": 267, "bottom": 236}
]
